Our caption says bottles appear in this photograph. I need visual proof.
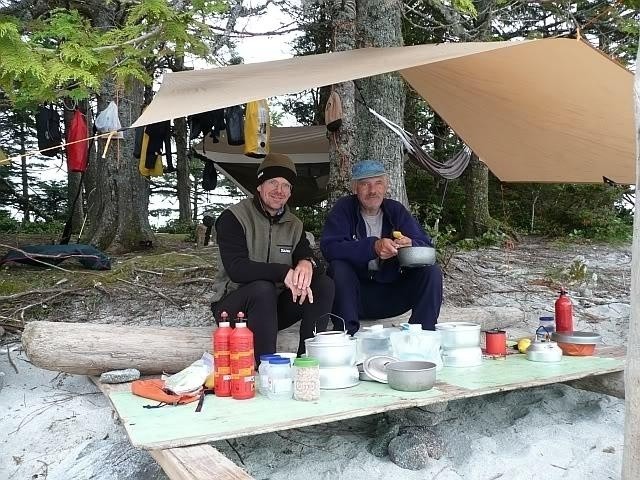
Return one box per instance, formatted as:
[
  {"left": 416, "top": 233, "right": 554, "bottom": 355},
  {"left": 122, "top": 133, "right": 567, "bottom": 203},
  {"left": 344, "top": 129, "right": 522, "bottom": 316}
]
[
  {"left": 214, "top": 310, "right": 320, "bottom": 402},
  {"left": 539, "top": 290, "right": 573, "bottom": 340}
]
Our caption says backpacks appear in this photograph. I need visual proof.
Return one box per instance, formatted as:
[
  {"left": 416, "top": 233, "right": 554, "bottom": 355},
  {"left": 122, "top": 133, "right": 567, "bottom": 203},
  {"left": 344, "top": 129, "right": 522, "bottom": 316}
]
[
  {"left": 243, "top": 99, "right": 271, "bottom": 159},
  {"left": 324, "top": 85, "right": 343, "bottom": 133},
  {"left": 35, "top": 102, "right": 63, "bottom": 162},
  {"left": 224, "top": 105, "right": 245, "bottom": 146}
]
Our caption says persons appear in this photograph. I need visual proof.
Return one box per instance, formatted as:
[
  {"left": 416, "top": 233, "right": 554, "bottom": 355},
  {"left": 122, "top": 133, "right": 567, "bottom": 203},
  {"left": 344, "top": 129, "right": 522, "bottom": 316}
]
[
  {"left": 210, "top": 152, "right": 335, "bottom": 372},
  {"left": 319, "top": 160, "right": 442, "bottom": 336}
]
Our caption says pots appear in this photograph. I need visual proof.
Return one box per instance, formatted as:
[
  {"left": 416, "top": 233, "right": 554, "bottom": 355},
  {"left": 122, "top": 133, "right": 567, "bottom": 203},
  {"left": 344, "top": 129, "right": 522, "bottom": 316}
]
[{"left": 304, "top": 312, "right": 483, "bottom": 392}]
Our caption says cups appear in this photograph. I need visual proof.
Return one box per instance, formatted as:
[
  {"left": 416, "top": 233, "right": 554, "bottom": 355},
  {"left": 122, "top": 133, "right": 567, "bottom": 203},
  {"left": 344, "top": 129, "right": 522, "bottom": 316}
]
[{"left": 486, "top": 330, "right": 507, "bottom": 354}]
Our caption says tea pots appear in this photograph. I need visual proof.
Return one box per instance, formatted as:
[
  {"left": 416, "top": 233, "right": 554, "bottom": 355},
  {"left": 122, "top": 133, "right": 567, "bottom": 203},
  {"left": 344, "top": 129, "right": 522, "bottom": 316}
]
[{"left": 525, "top": 326, "right": 563, "bottom": 362}]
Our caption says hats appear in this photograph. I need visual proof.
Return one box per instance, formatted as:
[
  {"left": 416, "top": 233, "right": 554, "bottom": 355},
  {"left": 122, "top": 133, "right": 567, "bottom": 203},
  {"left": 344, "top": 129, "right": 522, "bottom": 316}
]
[
  {"left": 350, "top": 159, "right": 387, "bottom": 181},
  {"left": 256, "top": 152, "right": 298, "bottom": 187}
]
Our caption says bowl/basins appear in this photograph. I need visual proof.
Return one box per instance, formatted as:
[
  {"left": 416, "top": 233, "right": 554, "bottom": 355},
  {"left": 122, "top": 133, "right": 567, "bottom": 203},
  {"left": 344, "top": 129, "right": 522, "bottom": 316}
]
[
  {"left": 553, "top": 331, "right": 601, "bottom": 355},
  {"left": 397, "top": 246, "right": 436, "bottom": 268}
]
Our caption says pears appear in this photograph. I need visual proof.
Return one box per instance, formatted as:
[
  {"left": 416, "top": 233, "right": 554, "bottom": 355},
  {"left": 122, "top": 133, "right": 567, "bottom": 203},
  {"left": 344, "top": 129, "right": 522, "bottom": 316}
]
[{"left": 518, "top": 338, "right": 531, "bottom": 353}]
